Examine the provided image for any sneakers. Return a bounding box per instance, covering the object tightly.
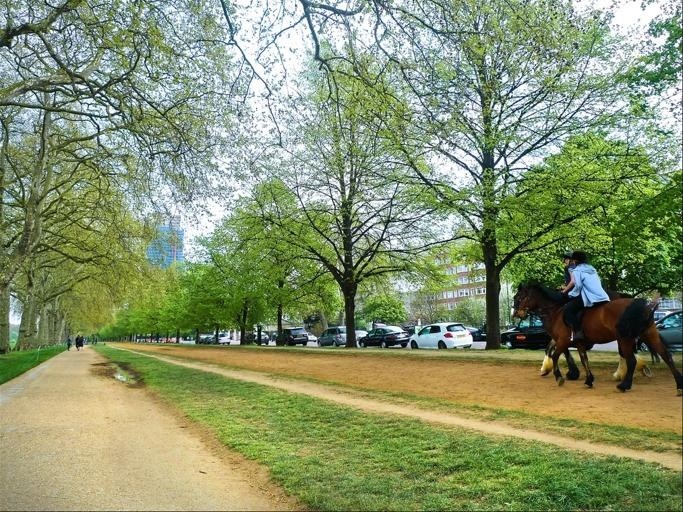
[573,330,585,339]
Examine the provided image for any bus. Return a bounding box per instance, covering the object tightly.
[136,334,151,344]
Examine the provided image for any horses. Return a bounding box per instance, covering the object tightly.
[512,276,683,396]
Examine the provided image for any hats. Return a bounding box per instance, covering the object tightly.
[563,251,586,260]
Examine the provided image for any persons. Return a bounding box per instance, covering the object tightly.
[561,251,576,286]
[63,334,71,351]
[74,334,80,351]
[560,250,611,342]
[80,335,83,347]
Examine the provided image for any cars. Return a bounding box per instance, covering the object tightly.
[637,308,683,352]
[193,332,231,345]
